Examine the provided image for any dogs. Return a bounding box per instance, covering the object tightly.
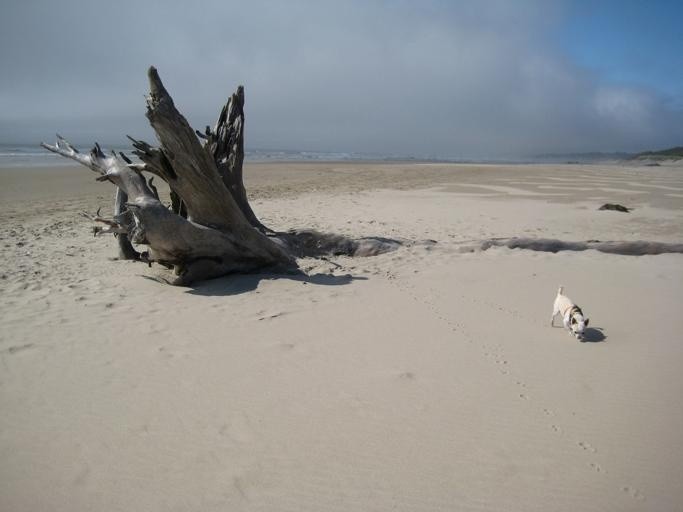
[550,285,589,340]
